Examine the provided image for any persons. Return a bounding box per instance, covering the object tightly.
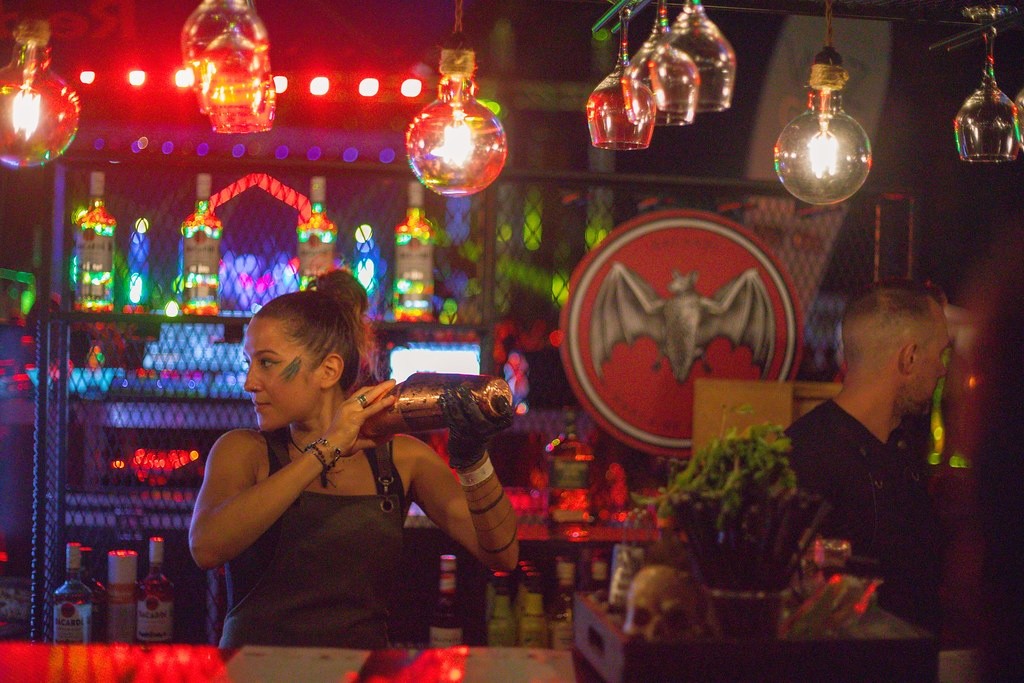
[774,279,948,602]
[189,268,519,647]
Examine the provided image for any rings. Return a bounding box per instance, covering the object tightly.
[355,394,367,404]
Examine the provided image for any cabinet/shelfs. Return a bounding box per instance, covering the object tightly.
[3,142,790,640]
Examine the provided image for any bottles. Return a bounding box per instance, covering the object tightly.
[180,173,222,315]
[73,171,115,315]
[483,553,610,651]
[52,537,176,645]
[392,181,434,322]
[428,554,465,649]
[549,408,591,525]
[296,176,339,294]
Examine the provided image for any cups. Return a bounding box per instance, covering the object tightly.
[1013,88,1024,152]
[359,372,513,439]
[701,583,792,639]
[180,0,276,133]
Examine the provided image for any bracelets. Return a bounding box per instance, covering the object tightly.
[305,438,344,488]
[459,457,494,487]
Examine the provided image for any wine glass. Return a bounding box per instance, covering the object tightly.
[586,0,737,152]
[953,4,1018,163]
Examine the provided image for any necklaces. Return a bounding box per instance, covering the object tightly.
[290,439,345,473]
